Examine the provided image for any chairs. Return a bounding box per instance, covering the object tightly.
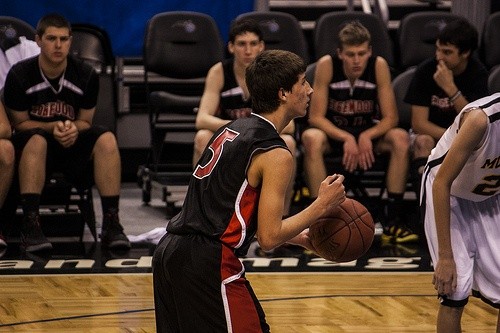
[0,16,118,242]
[139,12,500,220]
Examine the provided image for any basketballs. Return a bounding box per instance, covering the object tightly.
[309,198,375,262]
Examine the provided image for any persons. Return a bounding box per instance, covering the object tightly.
[152,50,345,333]
[0,100,15,259]
[301,21,418,242]
[420,92,500,333]
[2,15,131,252]
[403,15,489,178]
[194,21,296,246]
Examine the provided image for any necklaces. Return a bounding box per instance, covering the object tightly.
[40,67,66,94]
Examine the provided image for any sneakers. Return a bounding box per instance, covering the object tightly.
[102,214,132,250]
[303,248,319,256]
[21,212,52,253]
[381,223,418,242]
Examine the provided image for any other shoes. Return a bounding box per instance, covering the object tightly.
[0,227,8,247]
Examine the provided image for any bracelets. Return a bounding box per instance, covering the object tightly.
[448,90,461,101]
